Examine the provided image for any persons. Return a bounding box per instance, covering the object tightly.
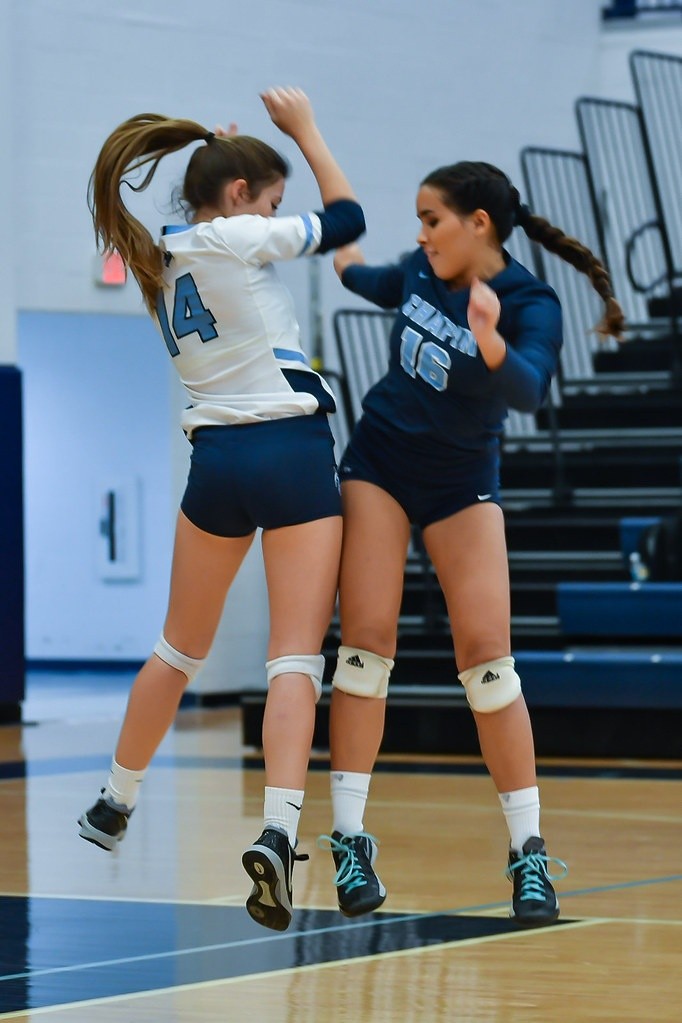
[75,85,366,933]
[317,160,627,926]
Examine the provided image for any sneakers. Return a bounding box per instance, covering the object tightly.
[505,836,567,928]
[77,788,135,851]
[316,831,386,918]
[242,825,309,932]
